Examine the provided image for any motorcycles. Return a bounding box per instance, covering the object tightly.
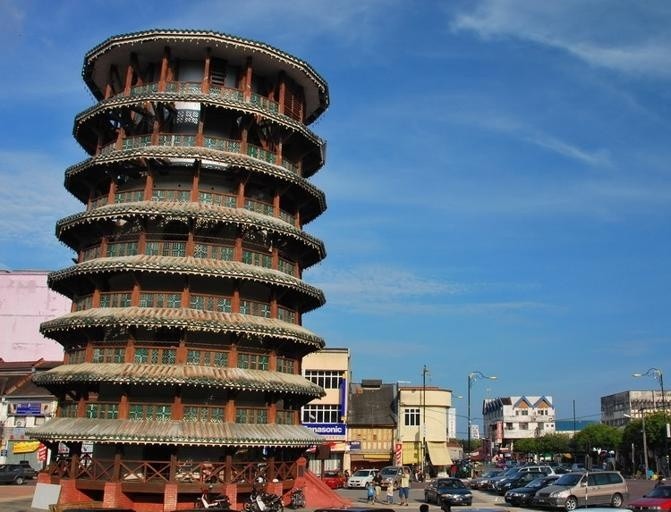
[243,481,307,512]
[194,485,232,509]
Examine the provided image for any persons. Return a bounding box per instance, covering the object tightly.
[345,466,423,506]
[419,504,429,512]
[441,500,452,512]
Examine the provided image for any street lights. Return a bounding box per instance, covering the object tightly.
[623,413,650,480]
[468,371,497,456]
[631,367,671,476]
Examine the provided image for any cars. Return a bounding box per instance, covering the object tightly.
[321,466,412,491]
[0,463,35,485]
[471,461,671,512]
[424,478,472,506]
